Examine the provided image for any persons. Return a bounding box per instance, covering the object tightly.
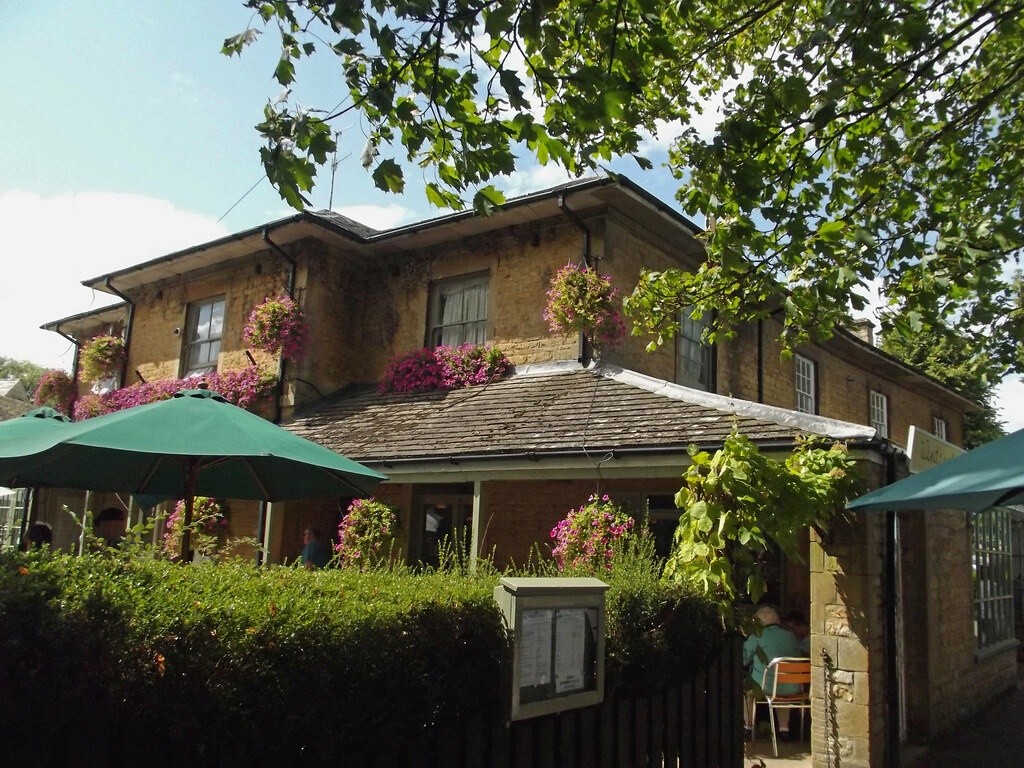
[302,526,327,569]
[93,508,126,550]
[785,611,810,657]
[742,606,802,738]
[23,522,53,550]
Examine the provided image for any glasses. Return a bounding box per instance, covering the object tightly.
[302,533,308,536]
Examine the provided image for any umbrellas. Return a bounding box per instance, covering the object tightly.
[0,407,72,444]
[0,377,391,559]
[846,428,1024,514]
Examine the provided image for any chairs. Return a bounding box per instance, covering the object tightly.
[751,657,812,757]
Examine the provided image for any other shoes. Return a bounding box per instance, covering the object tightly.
[743,728,751,741]
[778,730,791,741]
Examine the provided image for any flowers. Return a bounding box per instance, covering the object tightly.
[332,497,400,559]
[163,496,226,560]
[242,294,314,365]
[542,257,626,355]
[80,334,127,385]
[73,365,279,412]
[551,492,637,574]
[377,342,513,394]
[32,368,77,417]
